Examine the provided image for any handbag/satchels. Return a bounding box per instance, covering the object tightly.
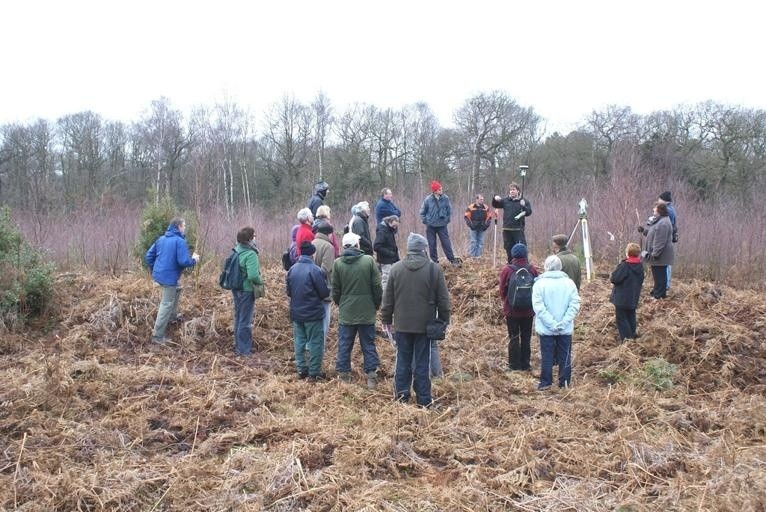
[640,250,650,261]
[426,318,446,340]
[253,285,265,298]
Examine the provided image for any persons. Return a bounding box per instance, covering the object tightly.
[463,193,492,257]
[637,202,675,300]
[492,182,533,263]
[498,242,540,372]
[531,254,582,391]
[229,226,266,357]
[419,180,457,265]
[284,182,403,391]
[380,232,450,409]
[551,233,582,293]
[658,191,679,291]
[608,242,645,344]
[143,218,202,343]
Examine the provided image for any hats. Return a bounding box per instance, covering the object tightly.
[319,223,333,234]
[660,191,674,202]
[552,234,569,245]
[431,180,441,193]
[407,233,427,251]
[299,241,316,255]
[543,254,563,270]
[511,244,528,257]
[342,232,361,248]
[316,181,328,192]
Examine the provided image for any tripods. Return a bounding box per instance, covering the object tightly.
[568,220,595,280]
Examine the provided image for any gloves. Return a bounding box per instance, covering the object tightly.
[638,226,644,232]
[552,322,565,331]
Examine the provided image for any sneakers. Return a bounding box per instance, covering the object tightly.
[298,371,326,383]
[368,369,393,380]
[151,337,170,344]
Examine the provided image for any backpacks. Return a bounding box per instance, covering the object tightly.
[282,245,295,270]
[507,263,534,307]
[219,248,249,290]
